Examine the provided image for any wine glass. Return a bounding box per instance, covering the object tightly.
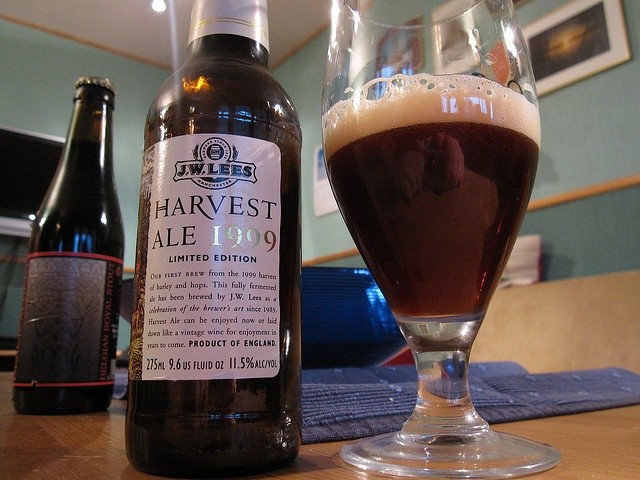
[320,0,561,479]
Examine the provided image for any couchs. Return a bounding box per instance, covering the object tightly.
[468,273,639,374]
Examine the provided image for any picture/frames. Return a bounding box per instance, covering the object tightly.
[521,1,630,98]
[374,18,423,90]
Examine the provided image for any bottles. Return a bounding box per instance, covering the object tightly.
[124,0,302,475]
[12,77,123,411]
